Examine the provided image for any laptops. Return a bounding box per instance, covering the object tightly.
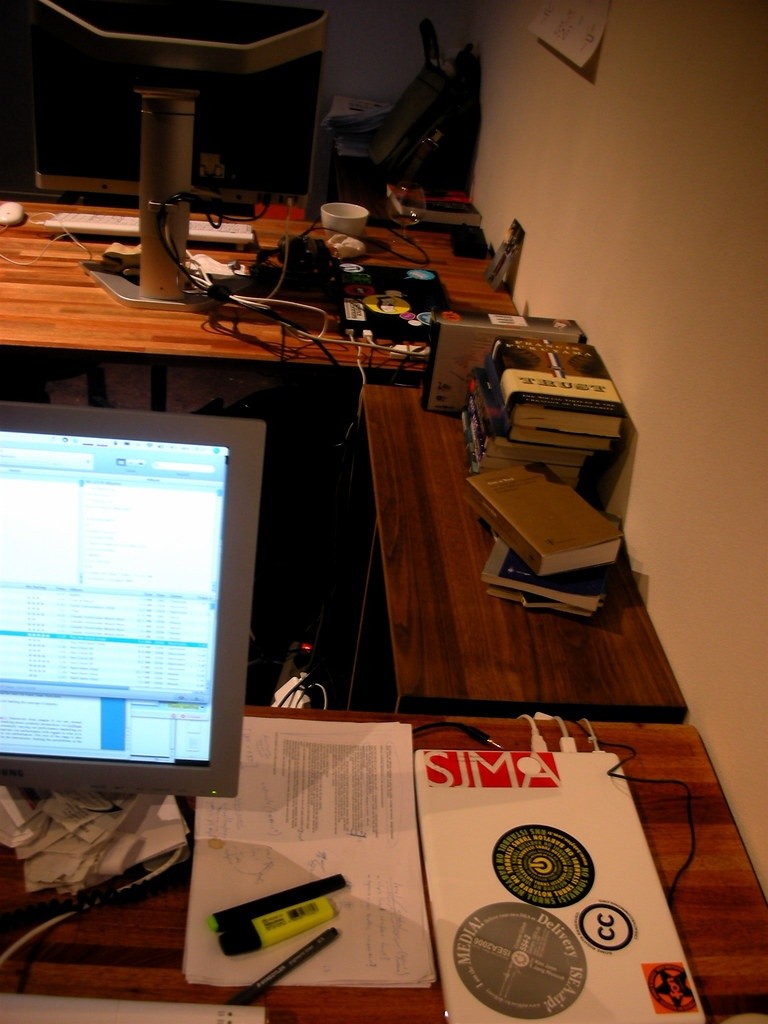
[336,264,453,340]
[410,749,706,1024]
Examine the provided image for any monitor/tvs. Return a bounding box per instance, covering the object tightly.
[27,0,329,311]
[0,402,266,799]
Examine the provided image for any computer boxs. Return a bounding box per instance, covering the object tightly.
[422,306,586,416]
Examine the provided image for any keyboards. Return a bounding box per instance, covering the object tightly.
[44,212,254,252]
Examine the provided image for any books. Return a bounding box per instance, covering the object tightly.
[387,181,482,227]
[460,333,630,618]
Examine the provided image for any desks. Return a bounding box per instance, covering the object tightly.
[345,383,687,719]
[0,706,768,1024]
[327,142,480,224]
[0,196,521,414]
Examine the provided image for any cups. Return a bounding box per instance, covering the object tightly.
[321,203,370,238]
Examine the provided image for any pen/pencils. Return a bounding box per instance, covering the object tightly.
[225,925,339,1005]
[207,874,346,932]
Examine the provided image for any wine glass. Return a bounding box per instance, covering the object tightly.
[386,182,426,248]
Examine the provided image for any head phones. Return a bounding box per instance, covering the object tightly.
[253,238,339,292]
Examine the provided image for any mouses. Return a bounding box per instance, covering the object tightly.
[0,202,24,226]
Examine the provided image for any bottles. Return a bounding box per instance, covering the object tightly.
[402,129,443,180]
